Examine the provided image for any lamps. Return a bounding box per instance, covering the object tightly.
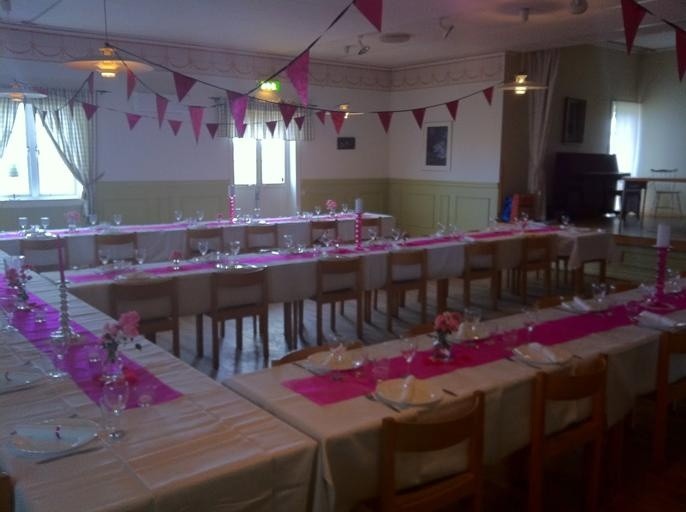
[494,6,549,96]
[356,34,371,56]
[1,80,47,104]
[568,1,589,14]
[438,16,456,39]
[62,0,155,79]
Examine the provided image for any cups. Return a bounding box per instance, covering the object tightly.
[135,382,156,408]
[33,306,47,323]
[86,342,102,361]
[11,255,24,273]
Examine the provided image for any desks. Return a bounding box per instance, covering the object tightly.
[620,175,685,230]
[2,210,685,511]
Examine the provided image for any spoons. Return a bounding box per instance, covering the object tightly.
[364,390,400,413]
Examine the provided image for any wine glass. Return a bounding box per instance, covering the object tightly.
[101,376,130,439]
[0,296,19,332]
[13,201,351,245]
[97,213,569,281]
[48,331,69,378]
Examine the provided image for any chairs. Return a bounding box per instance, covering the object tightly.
[648,167,682,218]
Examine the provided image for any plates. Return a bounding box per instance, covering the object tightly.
[6,418,101,454]
[305,269,686,407]
[0,366,46,391]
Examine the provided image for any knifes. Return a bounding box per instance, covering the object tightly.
[292,360,323,376]
[36,447,102,463]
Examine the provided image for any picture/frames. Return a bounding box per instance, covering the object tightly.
[420,120,452,172]
[560,95,586,145]
